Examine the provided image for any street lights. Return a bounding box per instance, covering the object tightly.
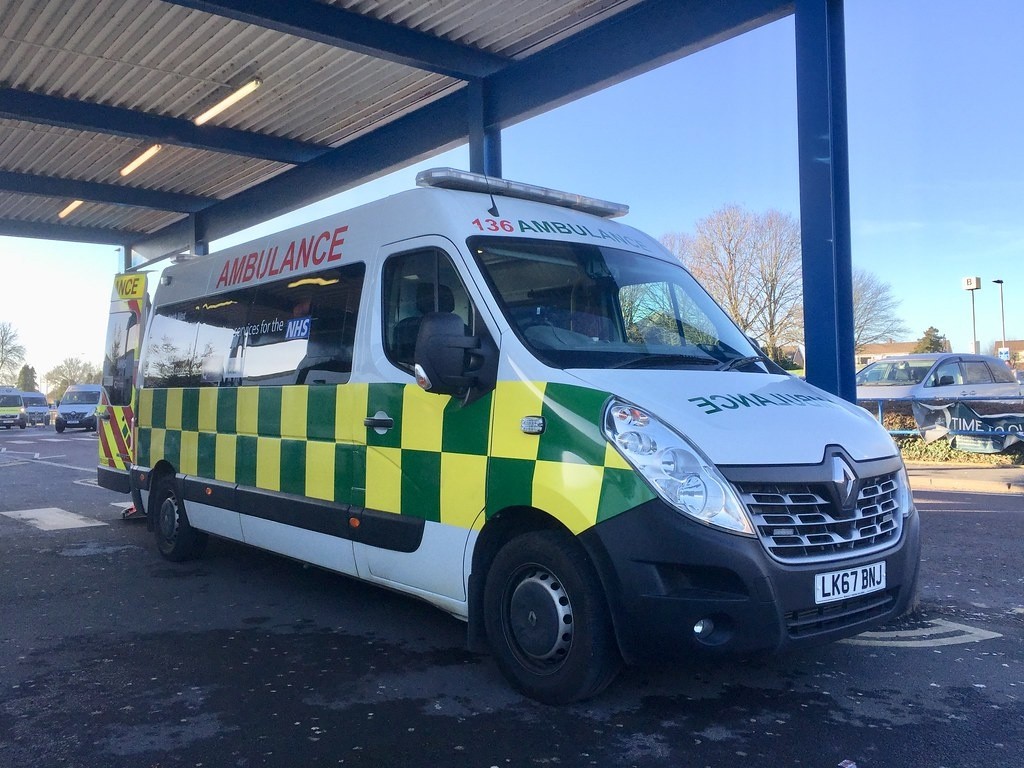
[993,279,1006,360]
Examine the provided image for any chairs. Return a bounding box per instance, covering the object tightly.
[894,370,911,380]
[936,366,954,378]
[513,300,563,327]
[73,396,79,401]
[393,282,474,378]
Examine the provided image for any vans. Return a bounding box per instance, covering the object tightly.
[20,391,51,426]
[54,384,102,433]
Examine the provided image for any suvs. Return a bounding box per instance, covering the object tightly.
[854,355,1024,439]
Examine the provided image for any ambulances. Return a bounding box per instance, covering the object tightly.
[93,164,923,707]
[1,385,27,429]
